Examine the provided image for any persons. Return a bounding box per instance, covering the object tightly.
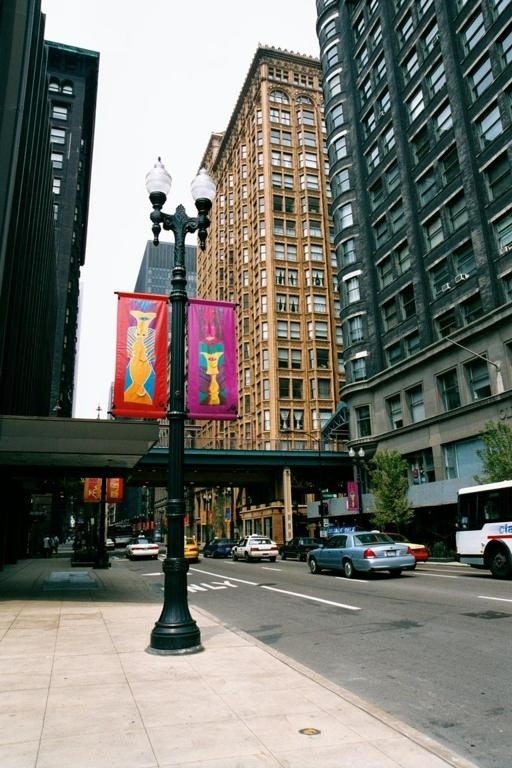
[43,536,60,557]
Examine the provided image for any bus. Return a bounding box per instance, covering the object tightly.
[454,479,512,579]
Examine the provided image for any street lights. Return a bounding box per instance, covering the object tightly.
[145,155,219,656]
[277,426,326,532]
[94,404,103,421]
[349,446,366,529]
[202,491,212,545]
[158,506,165,542]
